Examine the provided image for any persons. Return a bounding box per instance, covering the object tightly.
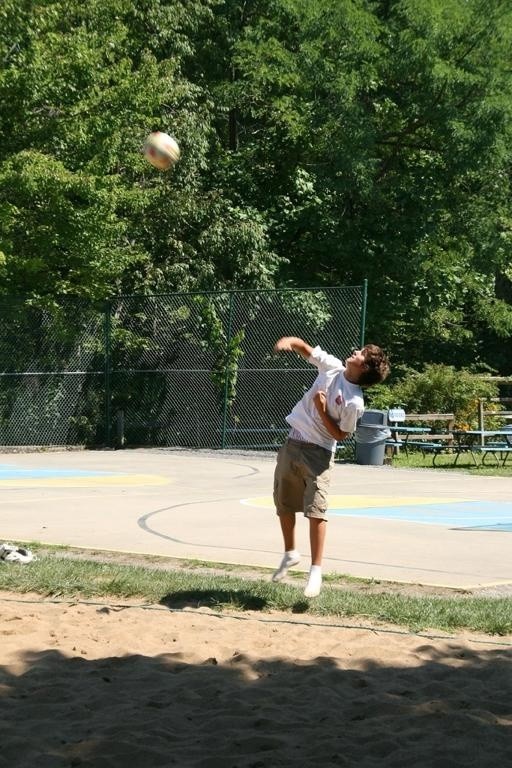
[271,336,390,598]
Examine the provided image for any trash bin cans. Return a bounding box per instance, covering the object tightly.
[354,410,391,465]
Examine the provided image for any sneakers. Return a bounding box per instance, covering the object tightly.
[0,542,33,564]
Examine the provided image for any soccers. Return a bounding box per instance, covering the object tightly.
[143,132,180,170]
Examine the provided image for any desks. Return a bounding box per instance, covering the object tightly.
[385,426,512,470]
